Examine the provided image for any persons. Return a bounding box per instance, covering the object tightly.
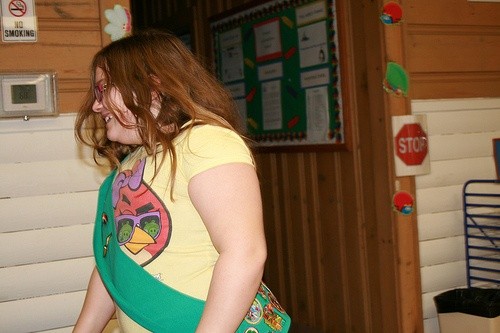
[72,28,292,333]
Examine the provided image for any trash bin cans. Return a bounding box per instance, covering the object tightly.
[433,287,500,333]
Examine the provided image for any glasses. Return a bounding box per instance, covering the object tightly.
[94,82,115,102]
